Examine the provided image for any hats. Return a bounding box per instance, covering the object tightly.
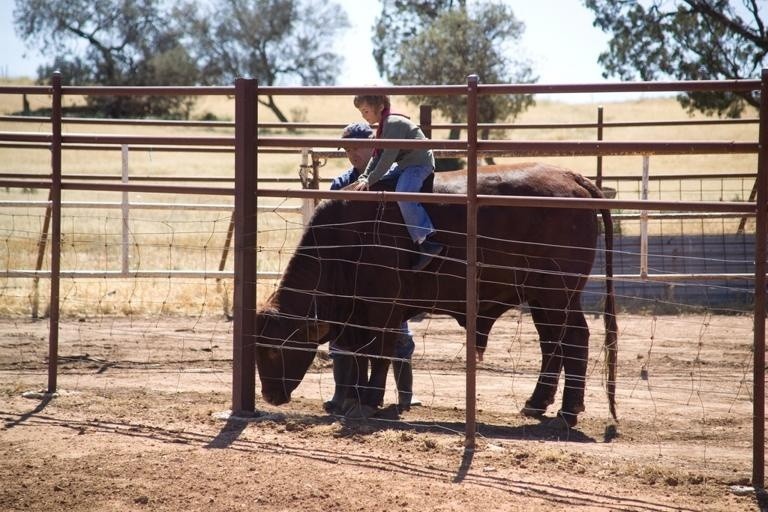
[338,122,376,150]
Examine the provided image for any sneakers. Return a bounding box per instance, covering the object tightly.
[412,239,443,272]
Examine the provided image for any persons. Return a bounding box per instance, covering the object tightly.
[324,122,416,412]
[353,94,446,272]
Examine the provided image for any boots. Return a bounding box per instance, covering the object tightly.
[323,357,354,413]
[387,359,413,413]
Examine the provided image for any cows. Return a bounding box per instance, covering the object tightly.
[256,161,619,430]
